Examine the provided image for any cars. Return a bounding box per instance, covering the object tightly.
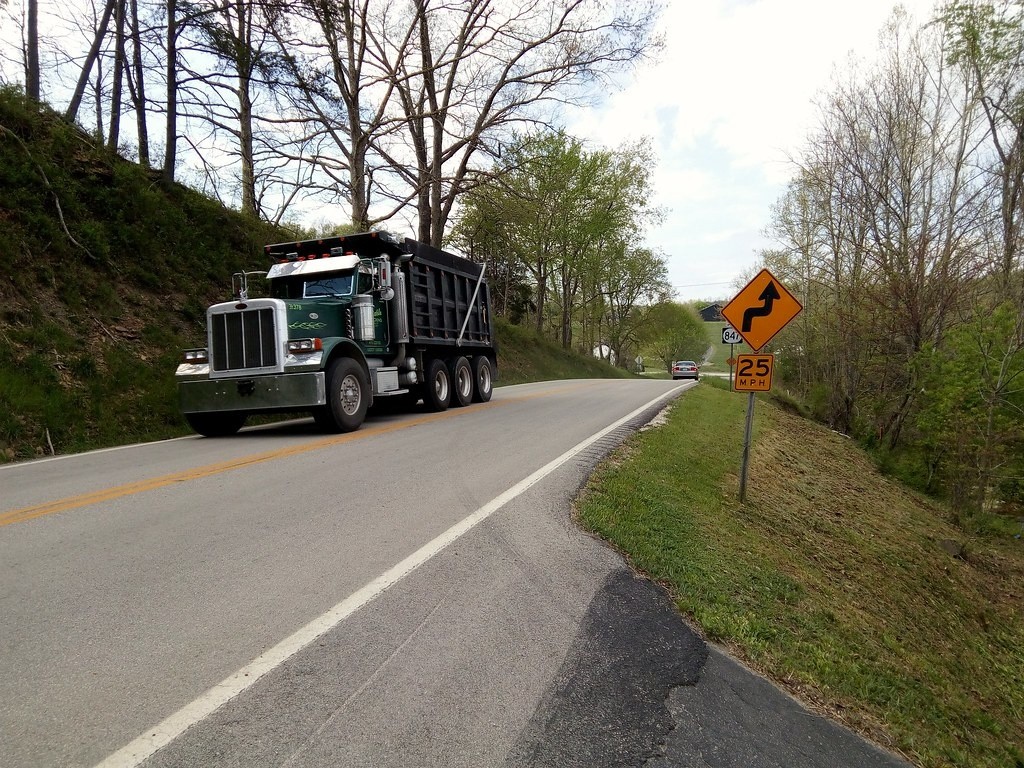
[672,360,700,381]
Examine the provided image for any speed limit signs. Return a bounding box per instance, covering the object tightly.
[734,354,774,393]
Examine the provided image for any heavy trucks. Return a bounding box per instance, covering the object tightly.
[170,230,500,439]
